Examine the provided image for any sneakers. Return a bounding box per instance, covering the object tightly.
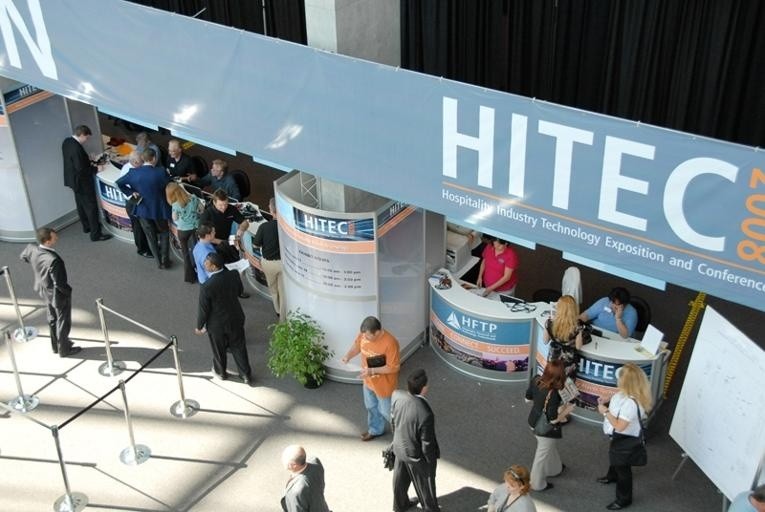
[361,433,384,441]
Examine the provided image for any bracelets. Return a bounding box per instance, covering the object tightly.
[602,411,610,416]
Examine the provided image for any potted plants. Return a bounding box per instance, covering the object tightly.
[260,305,337,389]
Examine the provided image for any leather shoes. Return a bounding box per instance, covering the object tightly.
[598,478,609,483]
[91,234,111,241]
[607,501,622,509]
[212,368,226,379]
[240,293,248,298]
[137,248,171,268]
[544,482,553,491]
[60,347,81,356]
[409,497,418,506]
[239,375,249,383]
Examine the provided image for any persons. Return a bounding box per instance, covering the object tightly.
[580,287,639,338]
[115,148,175,269]
[281,442,329,512]
[542,296,581,425]
[18,227,81,358]
[203,188,249,297]
[61,124,112,240]
[187,158,242,198]
[476,238,517,297]
[340,316,400,442]
[254,197,283,317]
[525,358,577,492]
[390,367,442,512]
[165,181,205,281]
[192,222,219,284]
[726,481,765,512]
[194,251,254,385]
[135,131,159,160]
[120,151,152,256]
[597,362,650,510]
[485,462,537,512]
[163,137,195,184]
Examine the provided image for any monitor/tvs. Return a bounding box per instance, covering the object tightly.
[499,292,525,305]
[181,182,203,198]
[110,159,123,170]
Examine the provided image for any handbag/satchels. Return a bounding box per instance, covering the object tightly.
[382,443,395,471]
[609,437,646,466]
[535,411,553,436]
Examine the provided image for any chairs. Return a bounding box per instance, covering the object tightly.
[531,289,561,304]
[629,296,653,331]
[156,144,251,203]
